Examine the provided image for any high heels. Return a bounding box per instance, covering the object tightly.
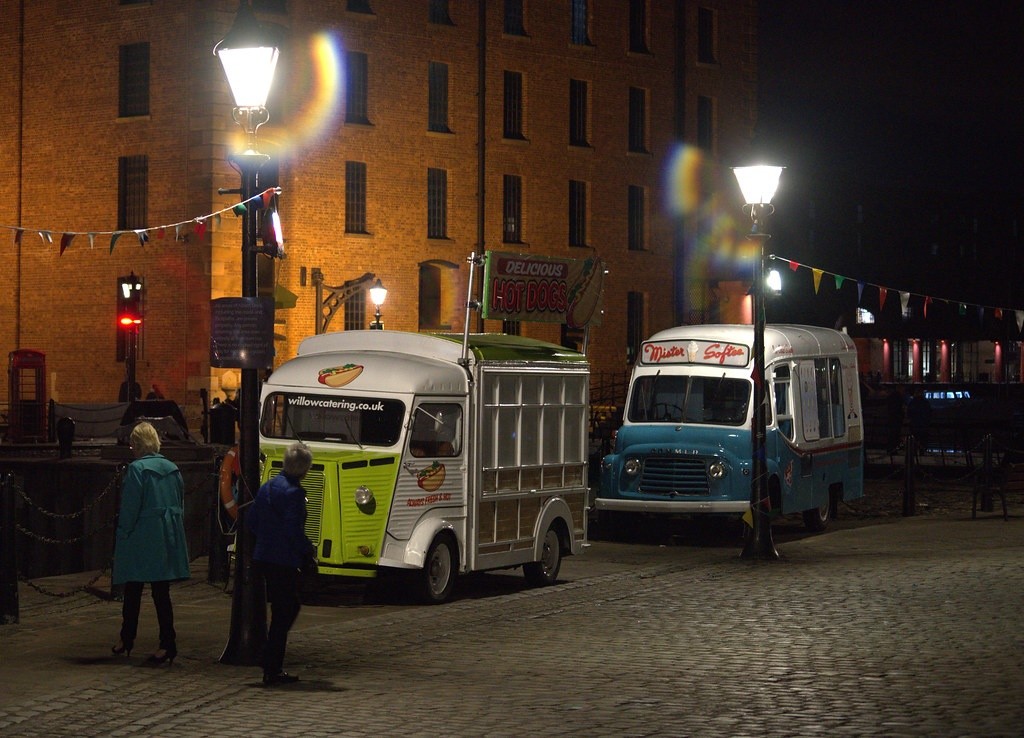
[111,641,134,656]
[147,648,177,664]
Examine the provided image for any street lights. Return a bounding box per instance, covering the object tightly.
[215,11,293,669]
[726,138,792,565]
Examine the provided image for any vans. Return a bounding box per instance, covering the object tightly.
[594,323,865,544]
[227,328,592,606]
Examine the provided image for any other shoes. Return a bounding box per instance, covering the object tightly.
[263,673,275,686]
[273,671,299,687]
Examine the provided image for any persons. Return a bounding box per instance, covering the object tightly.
[886,384,906,449]
[859,370,883,385]
[245,443,316,685]
[110,423,191,668]
[146,384,166,401]
[907,387,932,456]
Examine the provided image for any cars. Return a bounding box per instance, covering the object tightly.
[857,379,888,401]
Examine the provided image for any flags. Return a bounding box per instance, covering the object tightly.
[788,260,1024,334]
[13,187,276,255]
